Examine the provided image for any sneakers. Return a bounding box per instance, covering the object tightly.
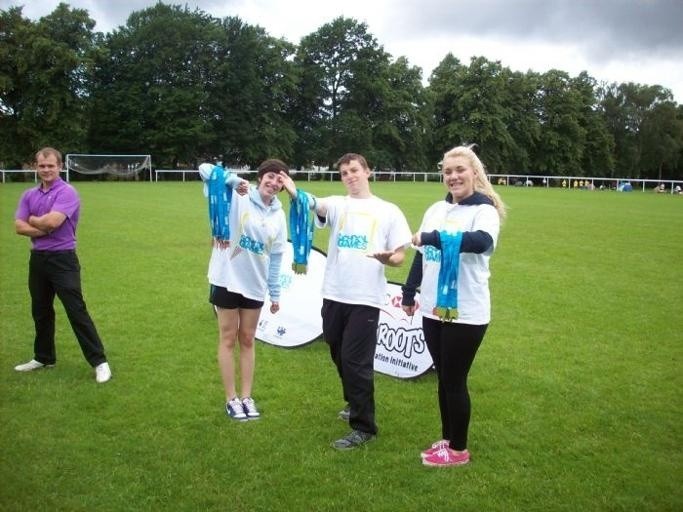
[95,362,111,383]
[240,397,260,419]
[14,359,54,371]
[336,403,351,423]
[421,447,469,467]
[420,439,450,457]
[329,430,376,450]
[224,397,248,421]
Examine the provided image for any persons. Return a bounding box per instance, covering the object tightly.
[277,151,414,451]
[197,158,290,422]
[653,184,667,193]
[674,185,682,193]
[495,178,590,188]
[400,144,511,467]
[12,147,112,384]
